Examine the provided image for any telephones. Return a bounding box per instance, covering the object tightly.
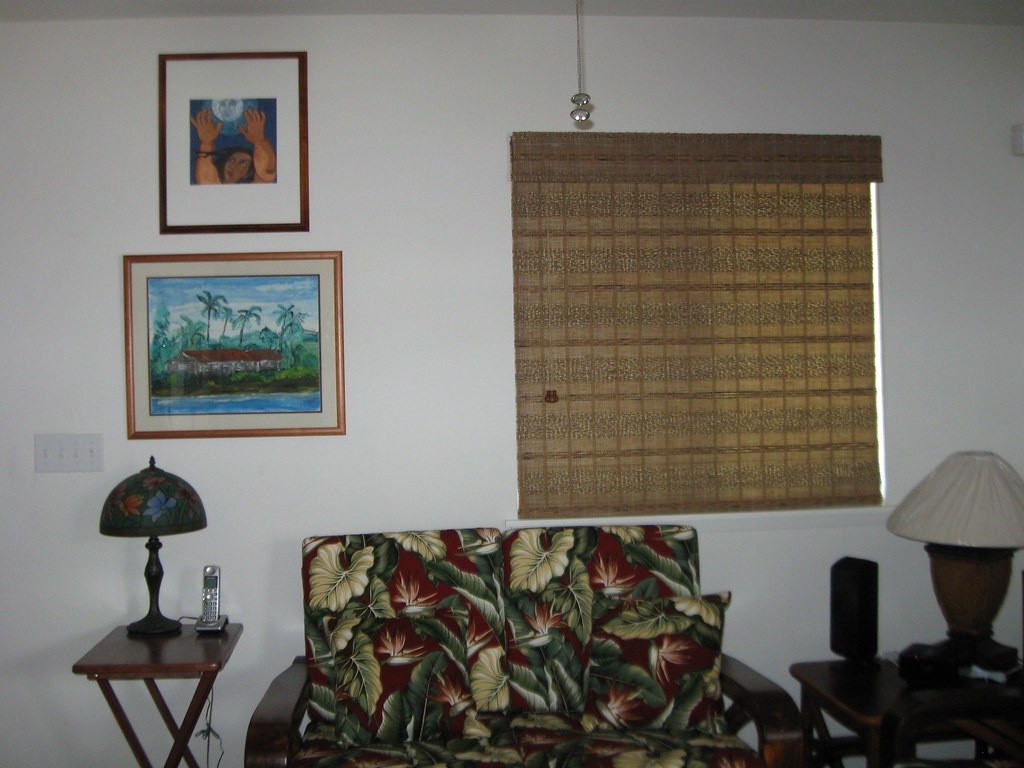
[194,564,229,634]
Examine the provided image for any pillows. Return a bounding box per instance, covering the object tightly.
[582,590,731,739]
[322,604,492,751]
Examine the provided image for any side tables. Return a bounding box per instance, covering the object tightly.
[789,650,1024,768]
[72,623,243,768]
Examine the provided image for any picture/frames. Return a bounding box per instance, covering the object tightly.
[159,50,309,235]
[123,250,346,440]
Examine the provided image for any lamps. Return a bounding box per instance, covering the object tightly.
[99,455,207,636]
[886,451,1024,671]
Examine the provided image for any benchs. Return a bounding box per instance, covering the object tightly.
[244,524,804,768]
